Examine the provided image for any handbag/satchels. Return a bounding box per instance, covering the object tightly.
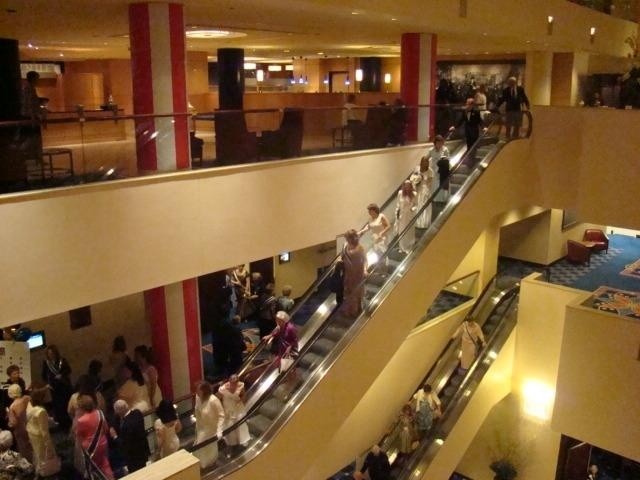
[328,271,344,293]
[477,337,483,347]
[265,334,295,373]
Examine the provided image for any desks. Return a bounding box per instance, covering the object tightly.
[26,147,74,182]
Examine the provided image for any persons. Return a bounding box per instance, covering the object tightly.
[336,229,370,319]
[352,442,392,479]
[25,70,49,149]
[215,373,252,448]
[448,97,489,169]
[341,94,361,145]
[0,326,163,480]
[356,202,390,274]
[394,179,419,256]
[436,78,498,136]
[425,133,451,206]
[209,264,304,387]
[364,100,387,124]
[618,67,639,109]
[450,316,487,370]
[191,379,225,470]
[385,402,421,455]
[409,155,434,229]
[153,399,182,461]
[495,76,530,138]
[405,384,444,433]
[587,464,603,480]
[386,98,406,115]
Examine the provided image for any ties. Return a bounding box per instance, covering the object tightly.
[512,89,515,97]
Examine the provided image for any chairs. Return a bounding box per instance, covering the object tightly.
[214,107,303,167]
[567,229,609,267]
